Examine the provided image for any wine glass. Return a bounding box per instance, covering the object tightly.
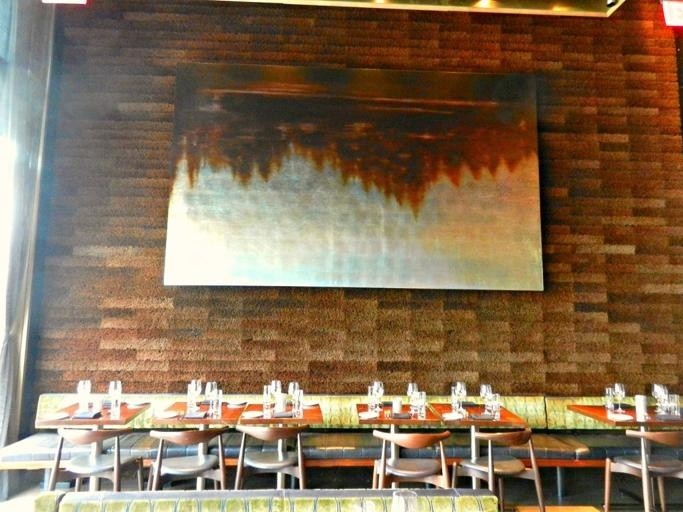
[606,384,683,416]
[74,379,305,420]
[369,379,501,422]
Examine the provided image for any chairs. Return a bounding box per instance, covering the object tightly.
[372,428,547,511]
[603,428,682,512]
[46,427,303,491]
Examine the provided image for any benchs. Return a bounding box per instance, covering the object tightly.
[34,489,499,511]
[546,395,683,458]
[1,396,575,469]
